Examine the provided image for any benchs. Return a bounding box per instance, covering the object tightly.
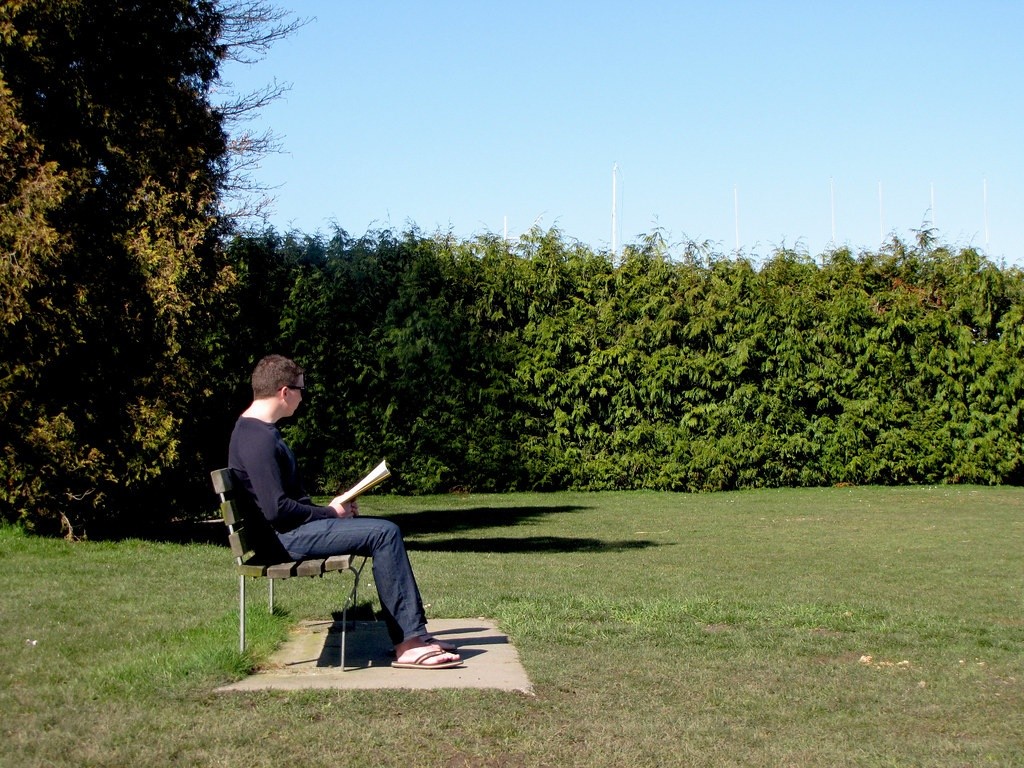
[211,468,367,672]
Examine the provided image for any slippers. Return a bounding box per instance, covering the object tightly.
[390,648,465,669]
[425,638,458,650]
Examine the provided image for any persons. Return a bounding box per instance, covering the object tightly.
[228,354,464,670]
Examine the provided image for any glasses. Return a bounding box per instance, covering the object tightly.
[278,385,306,399]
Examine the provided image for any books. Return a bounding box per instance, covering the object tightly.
[328,459,390,505]
